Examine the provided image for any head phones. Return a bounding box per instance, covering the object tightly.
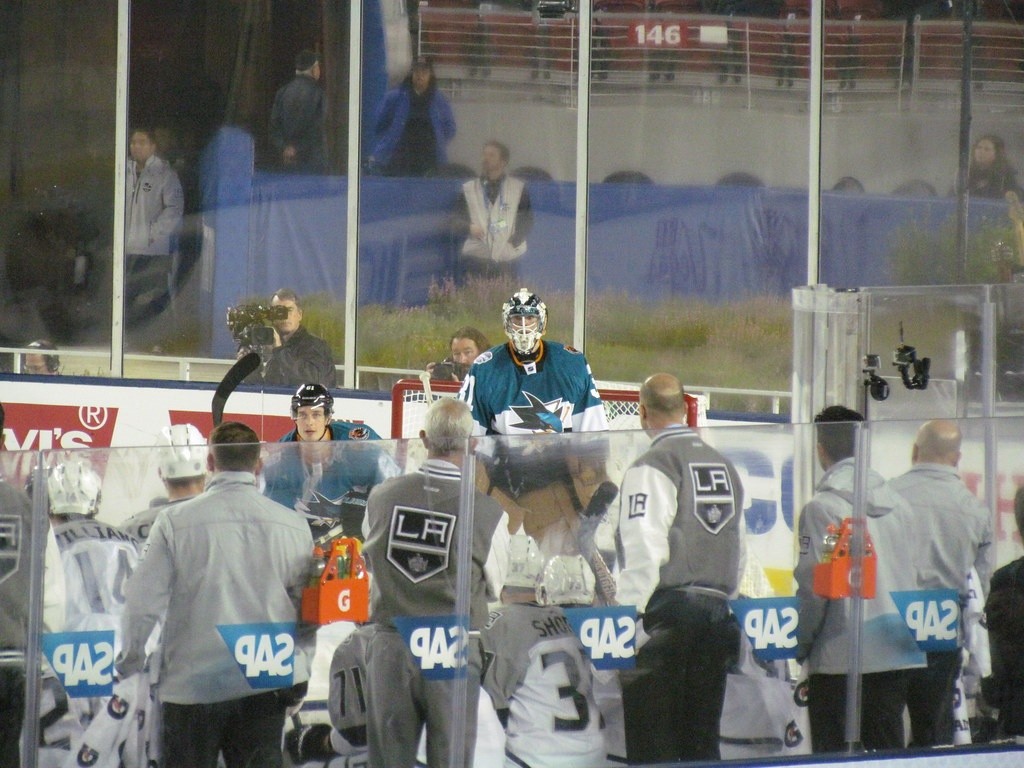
[35,338,60,373]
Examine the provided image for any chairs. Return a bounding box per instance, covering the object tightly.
[468,0,954,90]
[429,164,938,200]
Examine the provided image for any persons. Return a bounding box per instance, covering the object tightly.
[984,488,1024,746]
[267,50,329,176]
[456,289,611,559]
[427,325,491,382]
[887,420,994,746]
[362,396,508,768]
[125,127,184,354]
[0,403,370,768]
[447,141,531,283]
[264,383,400,547]
[616,374,751,765]
[482,535,607,768]
[238,288,336,388]
[363,57,456,176]
[793,406,929,754]
[24,340,60,375]
[948,133,1024,202]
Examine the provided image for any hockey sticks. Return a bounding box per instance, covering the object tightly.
[574,479,618,609]
[211,351,262,427]
[419,371,433,407]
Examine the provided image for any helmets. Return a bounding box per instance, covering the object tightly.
[157,423,209,478]
[292,384,334,414]
[501,288,548,356]
[47,463,101,515]
[536,555,596,605]
[503,534,542,588]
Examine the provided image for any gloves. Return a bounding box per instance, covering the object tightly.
[340,491,369,542]
[285,723,337,765]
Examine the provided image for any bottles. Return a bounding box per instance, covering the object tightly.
[308,536,366,589]
[822,524,872,561]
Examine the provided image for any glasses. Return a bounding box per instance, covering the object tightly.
[23,360,51,374]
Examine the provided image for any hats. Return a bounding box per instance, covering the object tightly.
[295,50,320,71]
[411,55,432,69]
